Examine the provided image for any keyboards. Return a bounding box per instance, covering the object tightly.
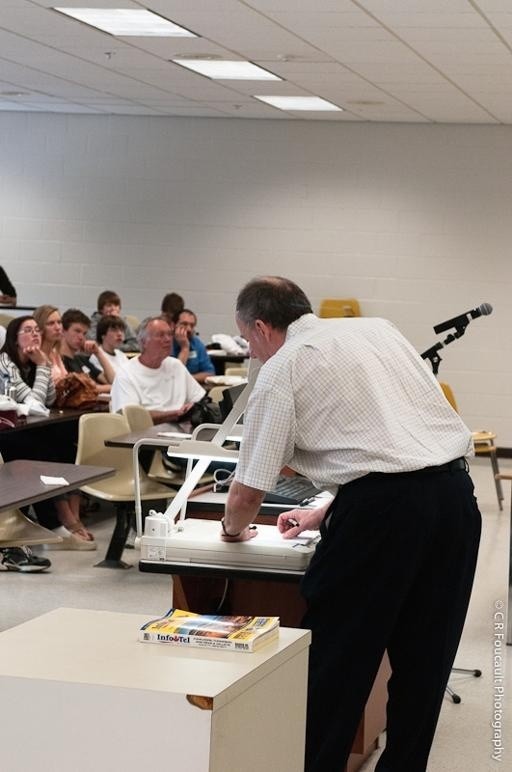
[263,474,320,505]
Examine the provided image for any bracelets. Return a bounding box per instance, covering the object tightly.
[219,517,242,537]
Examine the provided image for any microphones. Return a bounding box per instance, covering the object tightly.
[433,302,493,334]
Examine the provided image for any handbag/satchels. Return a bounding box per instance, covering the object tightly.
[190,402,222,428]
[55,372,98,409]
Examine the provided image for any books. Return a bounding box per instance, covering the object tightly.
[137,606,281,652]
[206,375,242,387]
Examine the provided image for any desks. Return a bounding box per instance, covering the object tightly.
[1,606,311,772]
[0,406,110,534]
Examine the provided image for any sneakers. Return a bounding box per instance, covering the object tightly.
[0,547,51,572]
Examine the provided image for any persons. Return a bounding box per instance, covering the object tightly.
[212,273,483,771]
[0,288,217,575]
[2,266,21,308]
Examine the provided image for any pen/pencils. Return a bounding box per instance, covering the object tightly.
[287,518,300,527]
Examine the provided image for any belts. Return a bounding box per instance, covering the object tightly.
[429,459,466,470]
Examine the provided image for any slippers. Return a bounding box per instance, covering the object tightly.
[67,523,94,541]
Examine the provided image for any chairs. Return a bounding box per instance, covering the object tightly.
[438,381,506,512]
[74,407,214,570]
